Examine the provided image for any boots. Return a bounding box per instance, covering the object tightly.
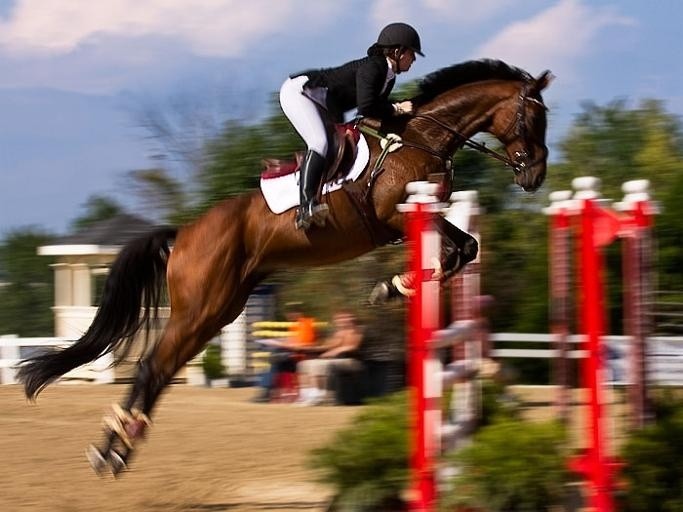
[295,150,329,233]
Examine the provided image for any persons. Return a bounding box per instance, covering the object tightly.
[279,23,424,230]
[248,302,364,403]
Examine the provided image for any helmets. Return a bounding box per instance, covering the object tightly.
[376,23,425,56]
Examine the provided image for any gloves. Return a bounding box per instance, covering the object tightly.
[391,101,415,115]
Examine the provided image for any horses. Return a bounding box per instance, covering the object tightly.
[11,56,556,482]
